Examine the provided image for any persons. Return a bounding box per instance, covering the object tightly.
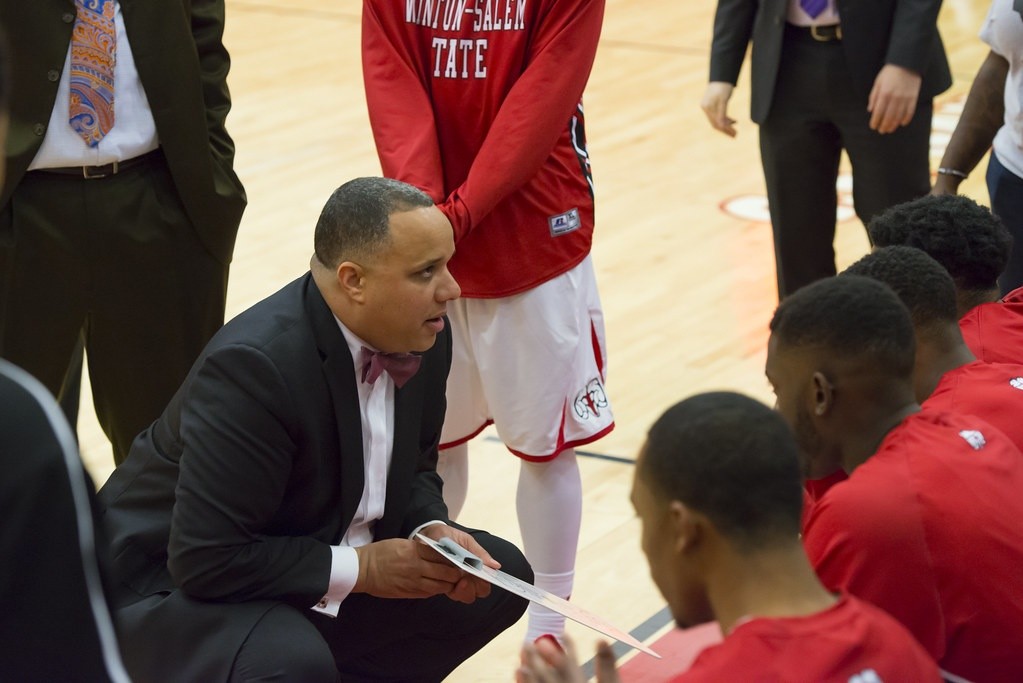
[507,392,945,683]
[0,0,249,490]
[767,276,1023,682]
[701,0,955,299]
[867,194,1023,367]
[1,359,135,683]
[930,0,1023,258]
[361,0,605,645]
[837,246,1023,453]
[94,178,533,683]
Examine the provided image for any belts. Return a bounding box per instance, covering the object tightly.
[41,147,164,179]
[780,19,844,43]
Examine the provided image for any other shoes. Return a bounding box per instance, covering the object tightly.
[533,633,565,655]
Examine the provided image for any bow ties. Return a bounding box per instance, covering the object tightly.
[360,346,423,388]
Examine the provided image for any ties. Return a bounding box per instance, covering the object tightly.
[68,0,115,147]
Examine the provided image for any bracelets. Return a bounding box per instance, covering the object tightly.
[937,167,967,177]
